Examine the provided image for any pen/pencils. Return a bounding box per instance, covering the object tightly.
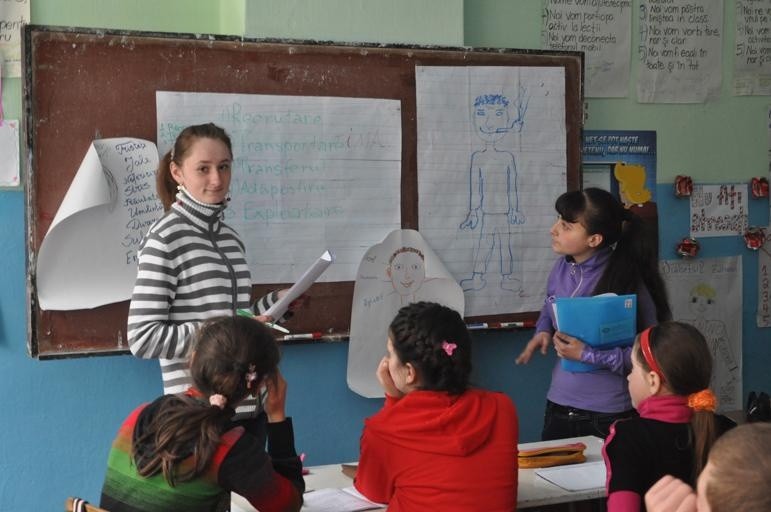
[235,308,290,335]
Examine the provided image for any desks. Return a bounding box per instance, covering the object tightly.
[229,434,606,512]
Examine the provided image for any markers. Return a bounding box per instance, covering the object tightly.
[496,322,533,328]
[465,323,488,330]
[284,332,321,340]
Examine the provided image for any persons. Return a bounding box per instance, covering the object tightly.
[647,423,767,511]
[126,123,311,396]
[100,317,305,512]
[352,302,517,512]
[515,187,660,442]
[602,322,738,511]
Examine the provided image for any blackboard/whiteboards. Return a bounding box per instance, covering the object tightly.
[20,24,584,360]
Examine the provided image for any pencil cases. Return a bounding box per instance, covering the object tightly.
[516,442,588,470]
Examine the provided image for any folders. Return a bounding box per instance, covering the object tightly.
[556,295,637,373]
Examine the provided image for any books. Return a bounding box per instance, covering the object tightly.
[536,460,607,492]
[302,487,388,512]
